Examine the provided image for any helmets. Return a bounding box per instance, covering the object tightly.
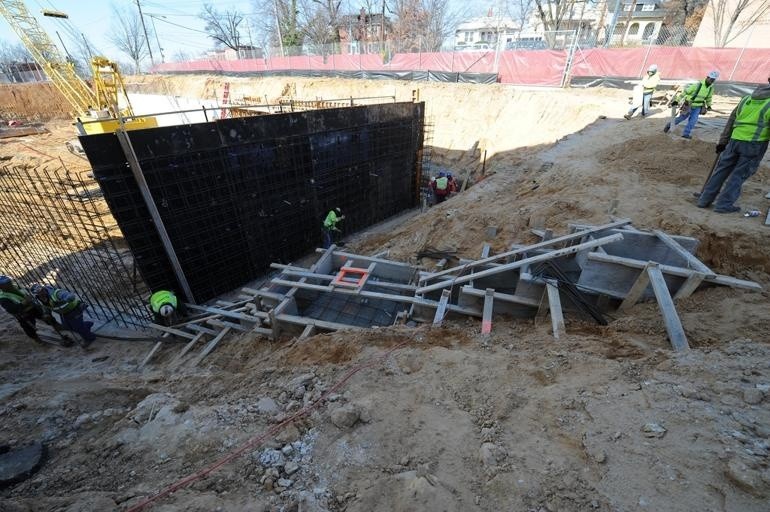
[647,65,657,75]
[708,71,718,79]
[31,285,45,297]
[0,276,10,284]
[440,172,451,176]
[160,305,173,317]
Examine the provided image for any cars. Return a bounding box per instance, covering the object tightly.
[454,38,591,53]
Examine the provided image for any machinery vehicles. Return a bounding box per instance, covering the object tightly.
[0,1,159,162]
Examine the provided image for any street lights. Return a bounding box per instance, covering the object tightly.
[151,14,168,62]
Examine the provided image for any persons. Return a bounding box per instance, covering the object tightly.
[149,289,180,326]
[431,171,452,202]
[30,282,95,349]
[663,71,718,138]
[0,276,59,347]
[446,173,458,192]
[323,205,346,245]
[698,78,769,214]
[625,64,660,119]
